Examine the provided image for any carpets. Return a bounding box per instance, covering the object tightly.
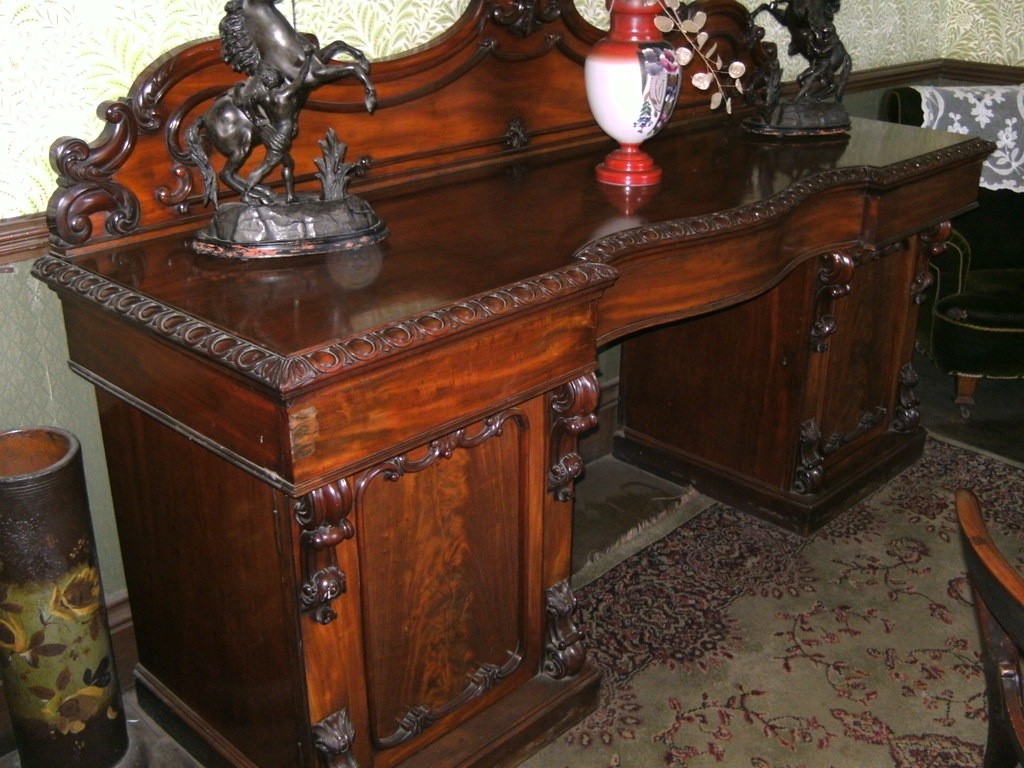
[517,430,1024,768]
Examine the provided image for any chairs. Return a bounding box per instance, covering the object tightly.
[956,488,1024,768]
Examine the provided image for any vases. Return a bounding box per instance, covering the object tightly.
[585,0,682,186]
[0,428,129,768]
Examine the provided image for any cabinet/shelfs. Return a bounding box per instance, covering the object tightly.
[28,0,997,768]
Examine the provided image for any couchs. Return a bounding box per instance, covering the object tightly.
[880,84,1024,418]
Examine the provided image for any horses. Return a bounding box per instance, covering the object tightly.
[745,0,853,105]
[182,0,380,212]
